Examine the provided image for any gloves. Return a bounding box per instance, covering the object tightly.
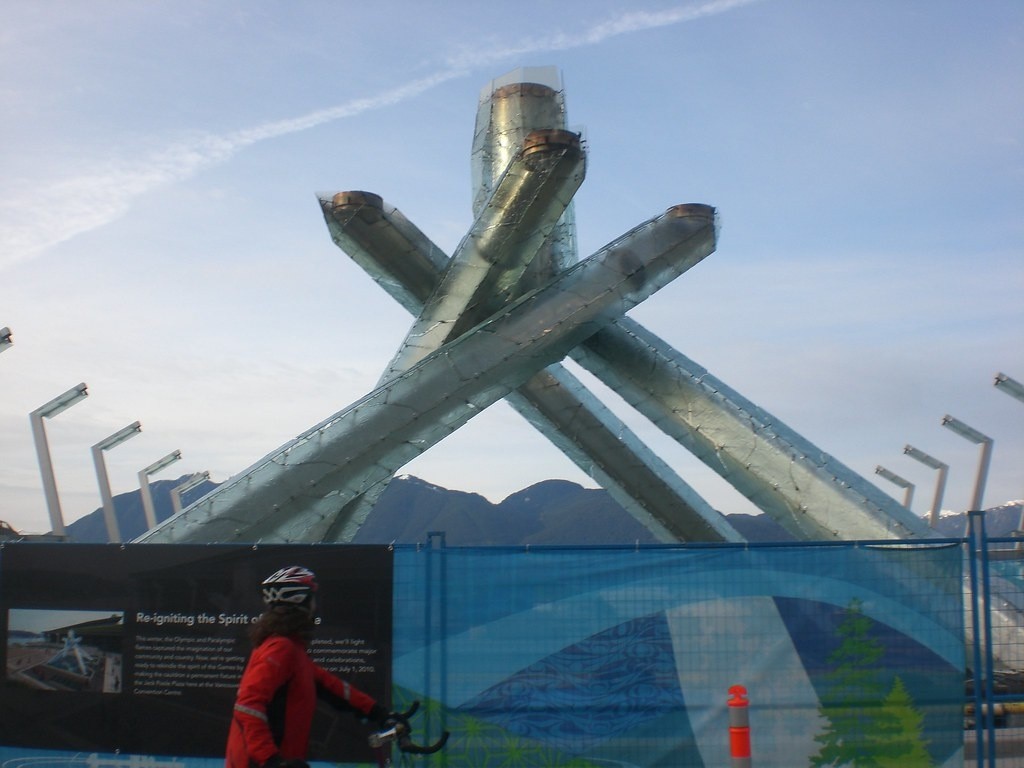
[263,753,288,768]
[367,703,391,724]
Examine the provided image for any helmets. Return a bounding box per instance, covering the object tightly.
[261,565,319,604]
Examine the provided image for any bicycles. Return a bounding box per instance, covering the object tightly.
[368,701,450,768]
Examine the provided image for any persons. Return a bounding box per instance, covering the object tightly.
[226,566,412,768]
